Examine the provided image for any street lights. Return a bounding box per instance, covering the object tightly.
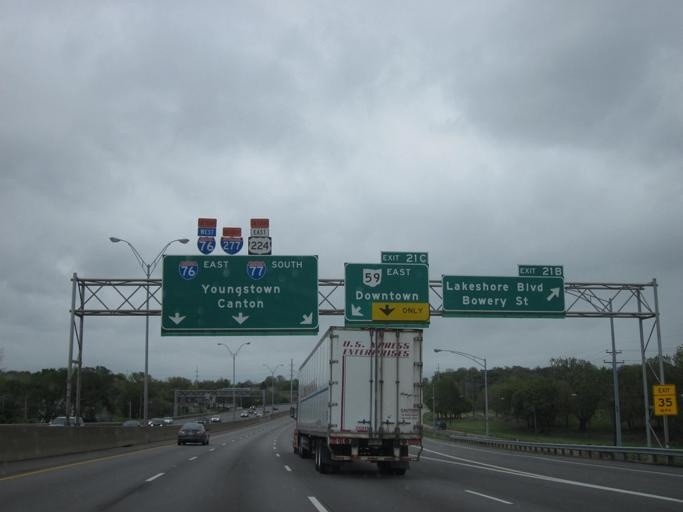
[107,231,191,427]
[433,346,491,438]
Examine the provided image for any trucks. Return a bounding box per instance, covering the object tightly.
[286,323,426,475]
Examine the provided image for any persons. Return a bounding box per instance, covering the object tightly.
[436,418,446,430]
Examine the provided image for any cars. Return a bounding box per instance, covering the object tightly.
[145,417,168,427]
[121,419,145,428]
[46,415,86,428]
[174,422,211,447]
[211,403,280,424]
[162,416,175,425]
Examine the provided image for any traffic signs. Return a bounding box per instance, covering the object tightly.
[260,362,284,414]
[343,250,430,326]
[441,265,565,319]
[197,216,218,255]
[161,254,319,335]
[246,216,274,255]
[220,225,246,255]
[215,340,251,422]
